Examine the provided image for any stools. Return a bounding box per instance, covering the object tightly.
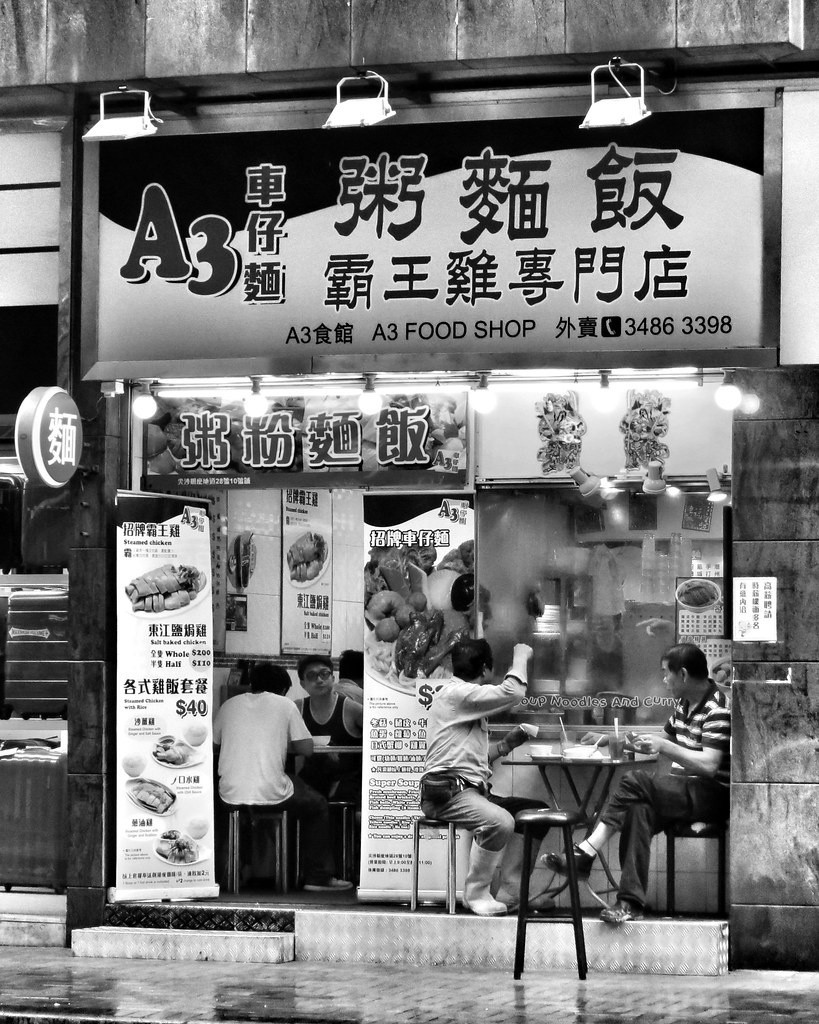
[514,807,587,982]
[220,800,358,897]
[409,817,474,914]
[663,822,727,917]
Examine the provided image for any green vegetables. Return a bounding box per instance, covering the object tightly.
[363,542,436,601]
[171,564,194,592]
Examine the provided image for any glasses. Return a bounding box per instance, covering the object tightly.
[304,669,332,680]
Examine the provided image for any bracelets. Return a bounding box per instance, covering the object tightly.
[497,740,508,757]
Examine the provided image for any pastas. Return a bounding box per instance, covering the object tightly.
[366,628,453,690]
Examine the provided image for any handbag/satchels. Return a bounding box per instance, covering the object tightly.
[422,773,467,802]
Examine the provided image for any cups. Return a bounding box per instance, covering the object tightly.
[639,533,692,601]
[609,733,625,760]
[560,731,576,754]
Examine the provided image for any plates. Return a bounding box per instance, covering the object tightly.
[524,753,562,759]
[365,655,417,697]
[153,830,210,865]
[533,605,561,639]
[285,533,330,587]
[121,573,208,618]
[126,778,182,817]
[532,678,588,693]
[151,735,205,768]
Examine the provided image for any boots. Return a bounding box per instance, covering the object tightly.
[494,833,557,911]
[463,837,507,916]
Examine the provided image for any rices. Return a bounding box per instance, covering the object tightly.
[185,721,206,738]
[123,750,146,768]
[186,817,207,839]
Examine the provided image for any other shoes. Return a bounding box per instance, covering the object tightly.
[600,900,644,924]
[303,877,353,891]
[541,844,596,881]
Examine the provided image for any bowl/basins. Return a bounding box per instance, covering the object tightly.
[674,579,721,613]
[123,763,146,777]
[529,744,553,756]
[312,736,332,745]
[186,824,208,840]
[182,728,207,747]
[710,656,732,693]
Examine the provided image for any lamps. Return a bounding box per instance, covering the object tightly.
[323,67,426,127]
[570,467,601,497]
[643,461,666,494]
[578,55,678,129]
[83,86,197,142]
[706,467,727,503]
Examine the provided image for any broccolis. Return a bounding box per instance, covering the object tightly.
[439,540,477,578]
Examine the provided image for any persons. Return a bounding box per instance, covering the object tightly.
[567,611,675,724]
[420,639,552,916]
[213,649,364,891]
[540,643,731,924]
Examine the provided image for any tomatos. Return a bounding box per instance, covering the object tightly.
[367,590,406,620]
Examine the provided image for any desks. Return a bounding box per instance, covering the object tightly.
[500,751,658,912]
[290,746,363,887]
